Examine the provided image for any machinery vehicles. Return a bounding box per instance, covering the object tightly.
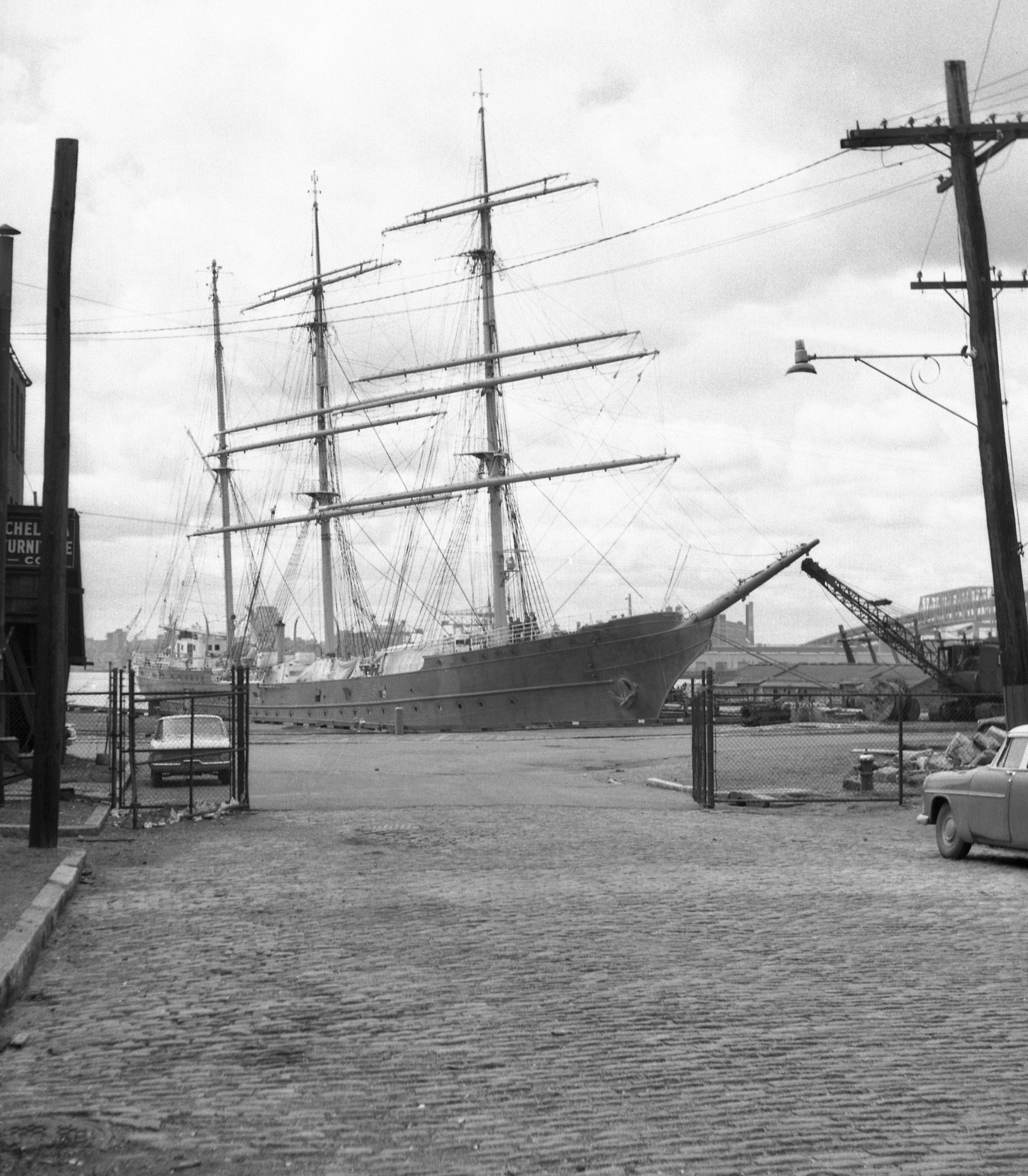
[802,557,1003,720]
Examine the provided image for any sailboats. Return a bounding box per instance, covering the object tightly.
[127,65,823,748]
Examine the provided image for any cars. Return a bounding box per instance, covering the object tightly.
[916,723,1028,862]
[144,713,232,787]
